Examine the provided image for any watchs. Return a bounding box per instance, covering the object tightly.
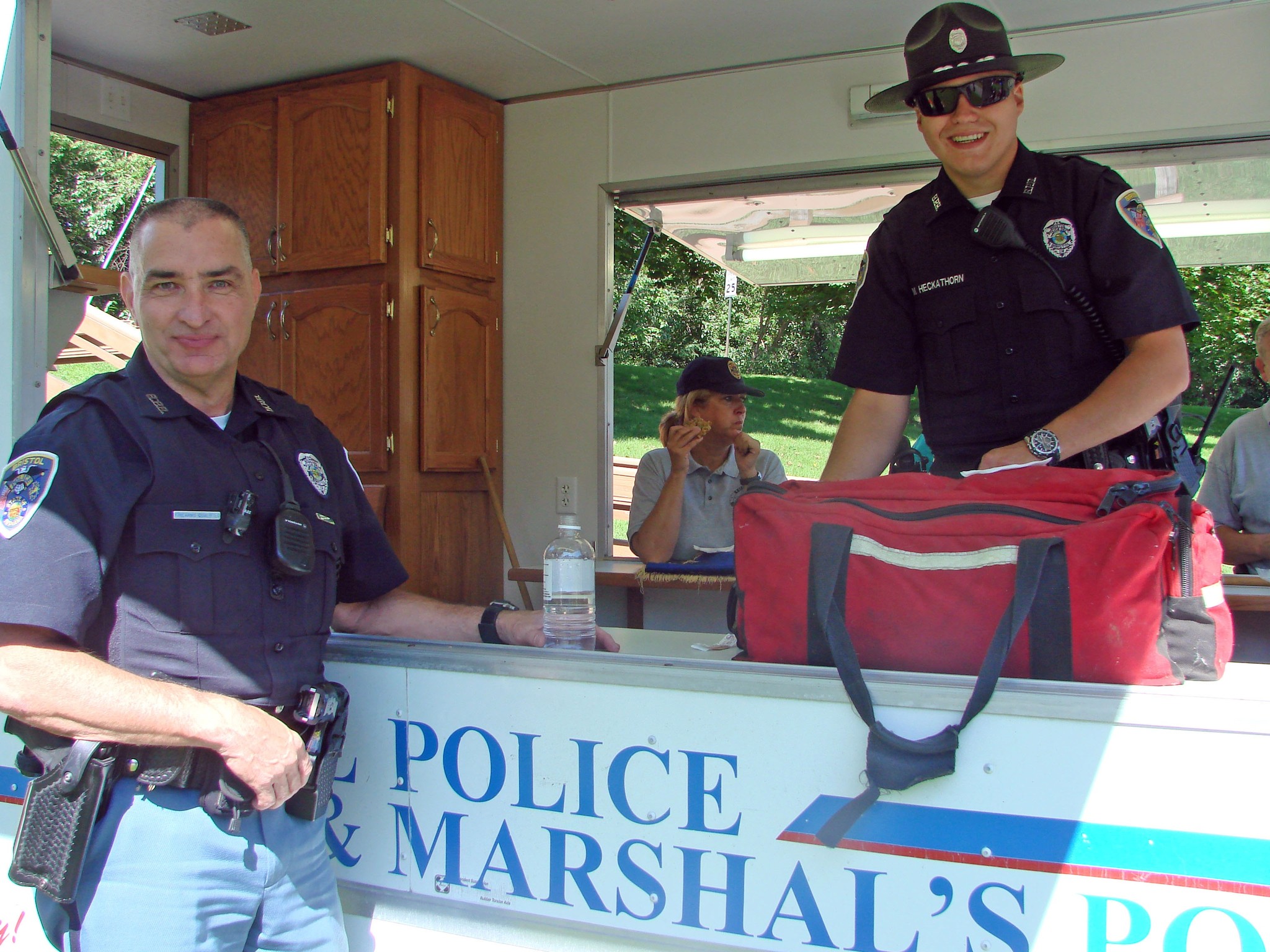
[739,471,763,486]
[1023,428,1062,465]
[478,600,520,643]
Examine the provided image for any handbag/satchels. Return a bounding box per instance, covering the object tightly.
[726,472,1235,791]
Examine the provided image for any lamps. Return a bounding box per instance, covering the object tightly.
[726,216,1270,262]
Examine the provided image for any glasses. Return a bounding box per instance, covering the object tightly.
[909,73,1024,118]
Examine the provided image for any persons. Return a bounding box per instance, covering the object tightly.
[627,354,788,565]
[815,3,1204,482]
[1195,315,1270,582]
[0,197,621,952]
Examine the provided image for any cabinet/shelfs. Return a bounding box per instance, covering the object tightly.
[184,60,505,608]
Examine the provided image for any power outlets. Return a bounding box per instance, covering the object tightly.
[556,475,578,515]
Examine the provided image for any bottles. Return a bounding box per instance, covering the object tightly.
[542,515,598,652]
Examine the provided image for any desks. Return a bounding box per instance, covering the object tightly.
[505,559,1270,629]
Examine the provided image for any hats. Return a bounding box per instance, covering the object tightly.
[866,2,1065,114]
[674,356,765,399]
